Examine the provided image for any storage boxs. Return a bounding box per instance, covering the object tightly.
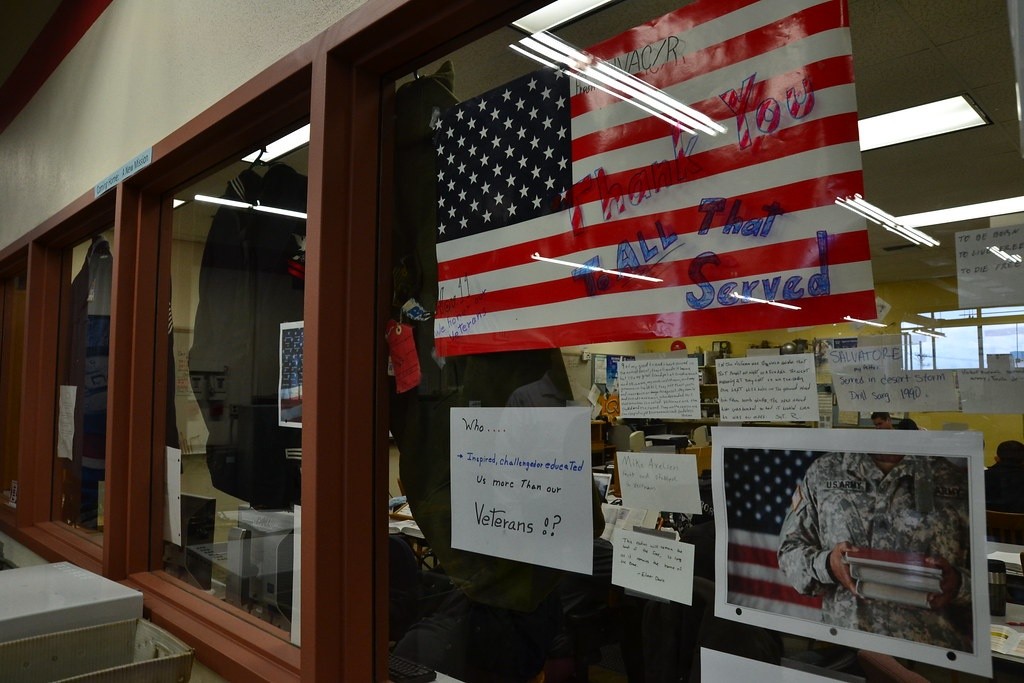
[0,561,195,683]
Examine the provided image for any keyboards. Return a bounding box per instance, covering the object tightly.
[388,653,437,683]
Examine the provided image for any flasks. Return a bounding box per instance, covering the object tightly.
[988,559,1006,616]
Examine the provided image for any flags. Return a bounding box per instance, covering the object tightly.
[435,0,878,359]
[725,447,975,652]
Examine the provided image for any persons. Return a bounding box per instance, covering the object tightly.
[493,329,616,512]
[868,410,1024,544]
[779,452,974,655]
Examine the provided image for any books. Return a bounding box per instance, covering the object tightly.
[845,550,944,609]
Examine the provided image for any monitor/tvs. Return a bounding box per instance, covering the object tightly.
[160,492,216,590]
[226,504,294,631]
